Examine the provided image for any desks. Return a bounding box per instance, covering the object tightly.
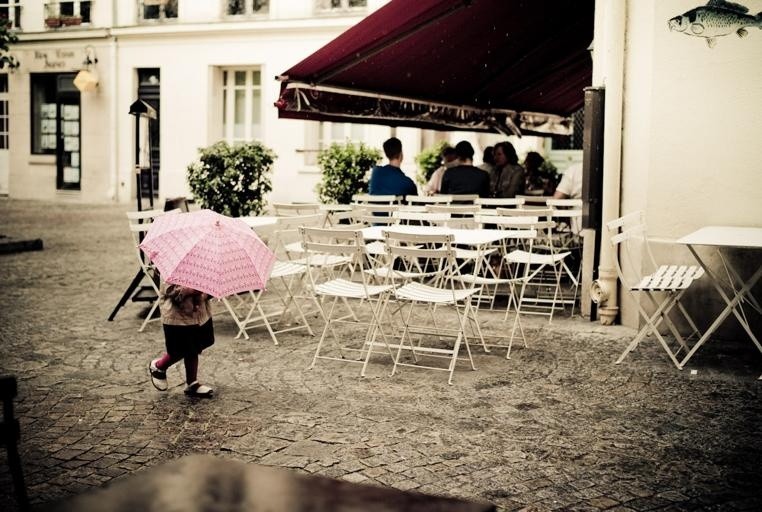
[676,226,761,368]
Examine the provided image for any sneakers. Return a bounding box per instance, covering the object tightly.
[148,359,170,391]
[185,381,213,395]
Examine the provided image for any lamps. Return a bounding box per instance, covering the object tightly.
[73,43,101,95]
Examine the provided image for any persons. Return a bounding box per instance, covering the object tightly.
[149,274,215,397]
[366,137,585,276]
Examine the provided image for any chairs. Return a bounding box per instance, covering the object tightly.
[107,190,585,386]
[607,210,702,370]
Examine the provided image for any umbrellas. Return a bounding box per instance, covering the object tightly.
[137,210,279,300]
